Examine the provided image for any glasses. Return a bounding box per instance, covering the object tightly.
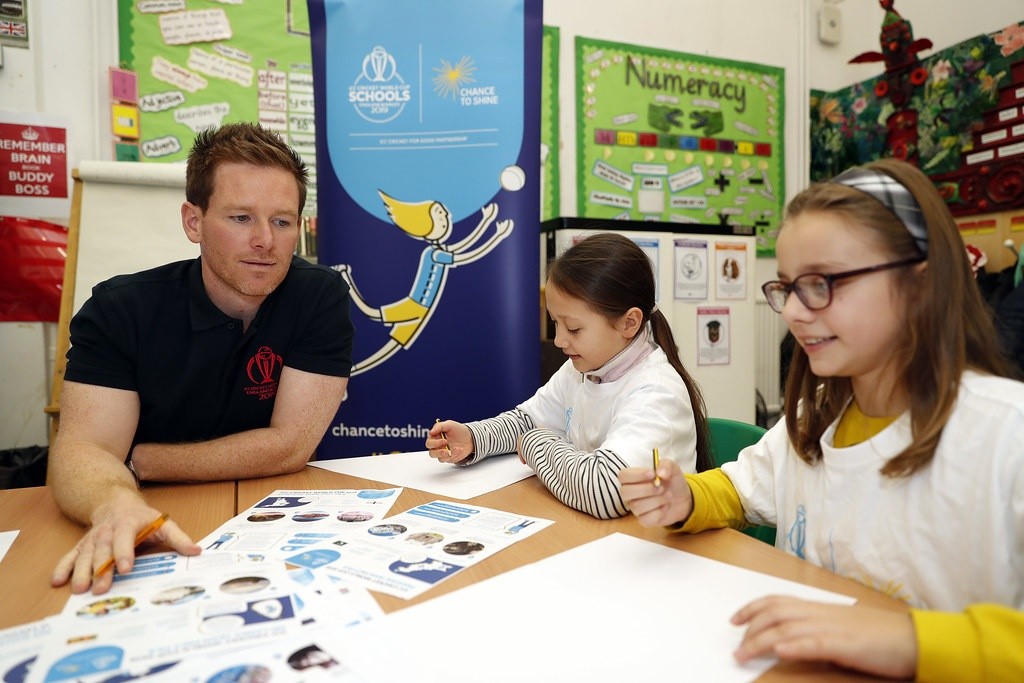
[761,255,930,314]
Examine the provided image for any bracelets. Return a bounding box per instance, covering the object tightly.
[125,443,138,488]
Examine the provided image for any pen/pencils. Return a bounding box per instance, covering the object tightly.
[652,448,661,488]
[436,418,452,458]
[90,511,170,582]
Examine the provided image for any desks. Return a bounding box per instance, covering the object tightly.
[0,465,920,683]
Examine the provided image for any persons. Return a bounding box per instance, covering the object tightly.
[618,159,1024,683]
[425,233,716,520]
[49,123,353,594]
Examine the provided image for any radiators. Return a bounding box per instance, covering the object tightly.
[755,299,788,415]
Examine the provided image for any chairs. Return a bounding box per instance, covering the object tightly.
[701,417,777,549]
